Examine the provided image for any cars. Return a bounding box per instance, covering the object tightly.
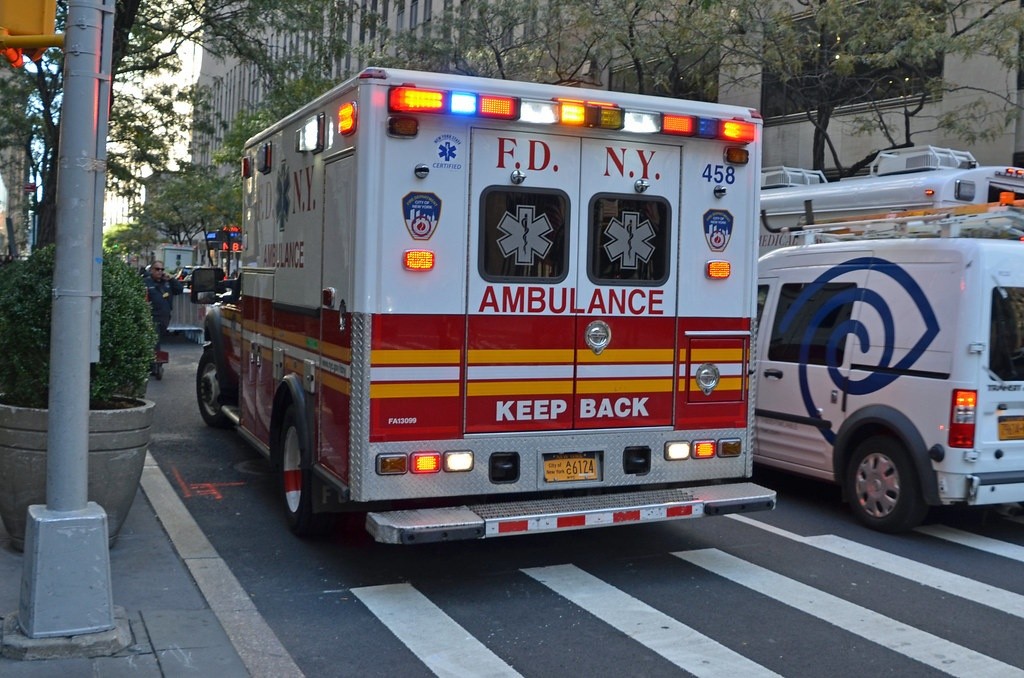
[171,263,226,289]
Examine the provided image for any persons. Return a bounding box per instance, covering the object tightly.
[144,261,184,380]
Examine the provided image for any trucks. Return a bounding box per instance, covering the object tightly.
[152,242,195,277]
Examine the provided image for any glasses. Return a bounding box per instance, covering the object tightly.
[151,267,165,271]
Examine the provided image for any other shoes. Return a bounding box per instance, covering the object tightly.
[151,363,158,374]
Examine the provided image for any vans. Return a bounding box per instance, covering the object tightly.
[754,143,1023,534]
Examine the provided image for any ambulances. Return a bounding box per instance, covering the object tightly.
[190,66,778,546]
